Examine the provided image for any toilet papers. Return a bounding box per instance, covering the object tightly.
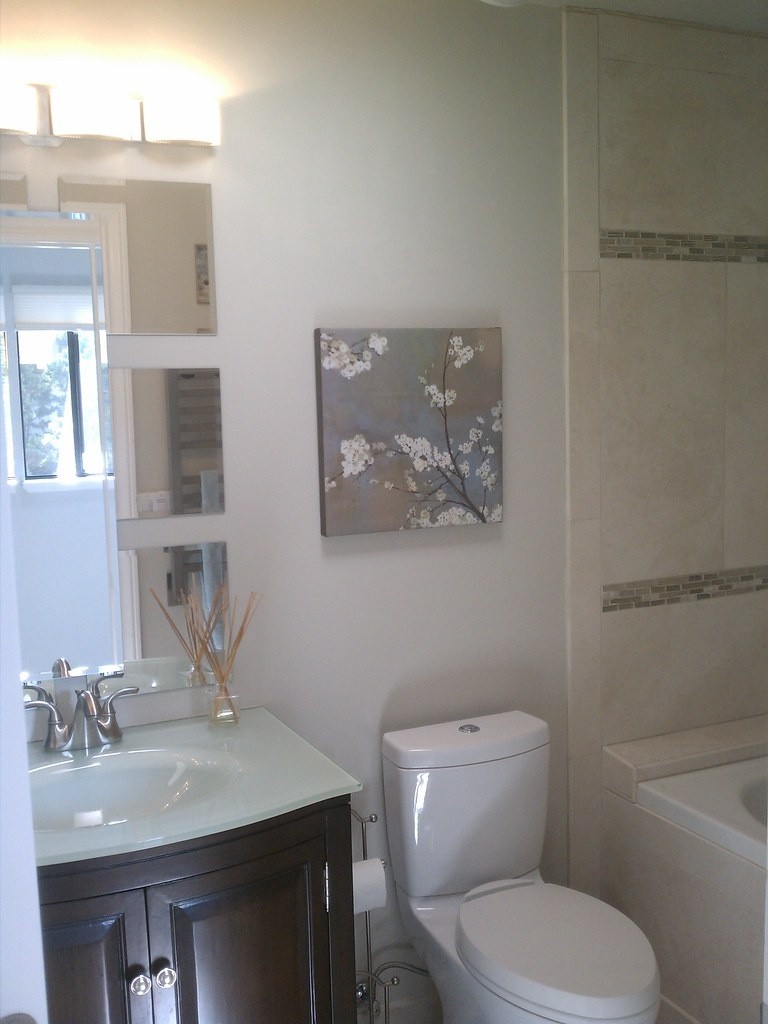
[352,857,387,916]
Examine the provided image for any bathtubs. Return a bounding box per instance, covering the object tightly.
[636,753,768,1024]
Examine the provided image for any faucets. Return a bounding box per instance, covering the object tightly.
[72,689,102,749]
[51,657,72,678]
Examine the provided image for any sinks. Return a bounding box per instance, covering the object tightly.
[28,749,242,832]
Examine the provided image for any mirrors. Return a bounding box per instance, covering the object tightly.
[0,178,221,709]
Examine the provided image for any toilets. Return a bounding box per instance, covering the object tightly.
[380,709,662,1024]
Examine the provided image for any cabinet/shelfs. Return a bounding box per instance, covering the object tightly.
[104,334,222,661]
[36,791,358,1024]
